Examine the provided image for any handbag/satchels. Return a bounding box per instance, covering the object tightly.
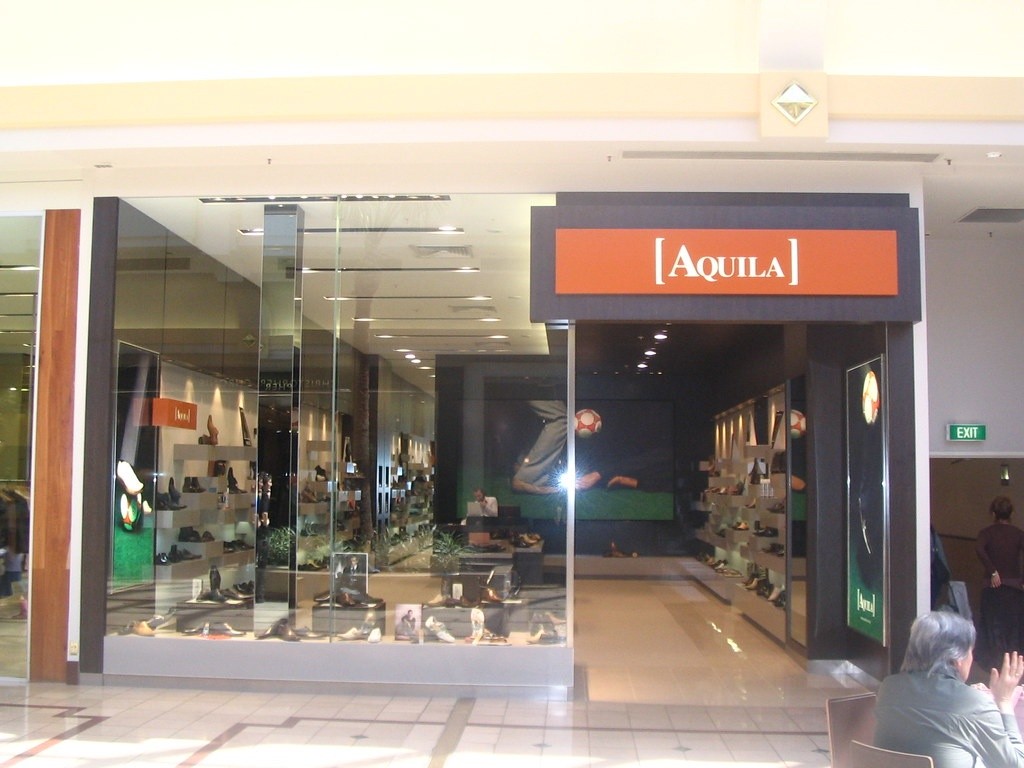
[946,579,972,622]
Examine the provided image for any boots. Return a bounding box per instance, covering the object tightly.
[12,599,28,620]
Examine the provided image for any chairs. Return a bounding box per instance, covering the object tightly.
[825,693,935,768]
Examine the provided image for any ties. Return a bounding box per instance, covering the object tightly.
[351,567,355,575]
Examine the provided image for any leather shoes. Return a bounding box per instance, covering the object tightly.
[474,527,541,553]
[208,414,218,445]
[228,467,238,491]
[429,586,504,608]
[157,525,255,567]
[156,476,206,510]
[254,617,381,643]
[313,587,383,608]
[695,454,787,611]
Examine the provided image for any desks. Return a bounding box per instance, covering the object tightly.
[457,540,546,585]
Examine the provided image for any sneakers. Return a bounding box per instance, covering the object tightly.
[131,620,155,636]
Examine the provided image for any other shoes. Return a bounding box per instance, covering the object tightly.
[184,621,243,636]
[197,565,254,602]
[391,471,435,546]
[301,463,366,553]
[603,549,626,558]
[426,615,567,645]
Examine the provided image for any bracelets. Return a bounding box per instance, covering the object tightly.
[992,571,999,576]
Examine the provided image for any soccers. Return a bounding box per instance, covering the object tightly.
[789,410,807,440]
[861,371,881,425]
[119,492,141,531]
[573,408,603,439]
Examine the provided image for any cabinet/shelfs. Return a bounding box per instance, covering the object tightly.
[152,441,434,605]
[693,444,806,647]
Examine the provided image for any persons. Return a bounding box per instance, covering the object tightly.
[0,545,28,620]
[973,497,1024,674]
[401,610,415,630]
[256,472,272,569]
[872,610,1024,768]
[931,526,951,609]
[473,488,498,517]
[511,400,668,495]
[343,556,366,592]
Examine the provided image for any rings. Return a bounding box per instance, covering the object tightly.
[1016,674,1020,676]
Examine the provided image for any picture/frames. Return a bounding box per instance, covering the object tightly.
[394,604,422,642]
[330,551,368,603]
